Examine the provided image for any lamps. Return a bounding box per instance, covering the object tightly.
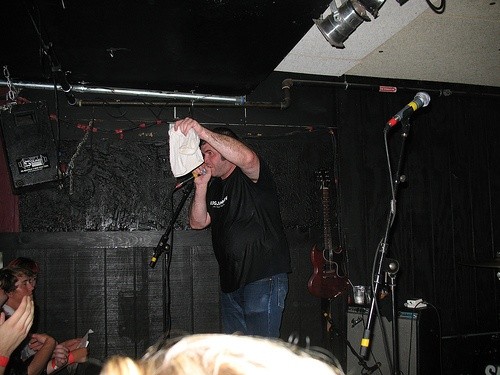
[312,0,408,47]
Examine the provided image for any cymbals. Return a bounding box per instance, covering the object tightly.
[462,256,499,269]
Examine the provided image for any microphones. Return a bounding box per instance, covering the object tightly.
[366,362,381,375]
[387,92,430,129]
[175,168,207,189]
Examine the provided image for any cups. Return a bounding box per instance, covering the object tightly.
[353,285,365,305]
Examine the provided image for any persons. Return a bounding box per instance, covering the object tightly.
[174,117,293,339]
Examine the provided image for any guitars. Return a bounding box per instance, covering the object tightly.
[303,167,366,313]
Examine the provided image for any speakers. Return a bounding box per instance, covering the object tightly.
[0,100,63,189]
[347,301,441,375]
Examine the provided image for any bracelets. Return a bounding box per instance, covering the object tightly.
[0,356,9,367]
[68,352,74,363]
[52,359,56,368]
[0,257,89,375]
